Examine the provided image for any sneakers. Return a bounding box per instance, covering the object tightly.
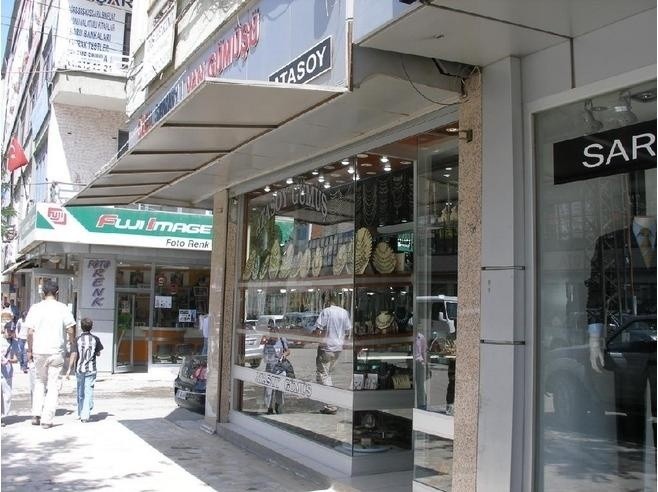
[267,403,280,415]
[32,416,52,428]
[81,419,87,423]
[319,407,337,415]
[1,418,6,427]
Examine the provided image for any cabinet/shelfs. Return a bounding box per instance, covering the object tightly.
[235,271,413,465]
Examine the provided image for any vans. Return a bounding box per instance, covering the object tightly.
[414,293,458,364]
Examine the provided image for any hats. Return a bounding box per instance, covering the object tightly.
[4,321,16,332]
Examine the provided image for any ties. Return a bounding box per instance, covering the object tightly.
[639,228,654,269]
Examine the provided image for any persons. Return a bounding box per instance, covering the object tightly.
[586,217,657,492]
[25,281,77,429]
[261,326,291,415]
[1,299,28,427]
[65,318,104,423]
[312,290,351,413]
[202,313,209,355]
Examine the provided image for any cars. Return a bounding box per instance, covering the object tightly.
[242,311,323,369]
[172,352,206,416]
[536,315,656,436]
